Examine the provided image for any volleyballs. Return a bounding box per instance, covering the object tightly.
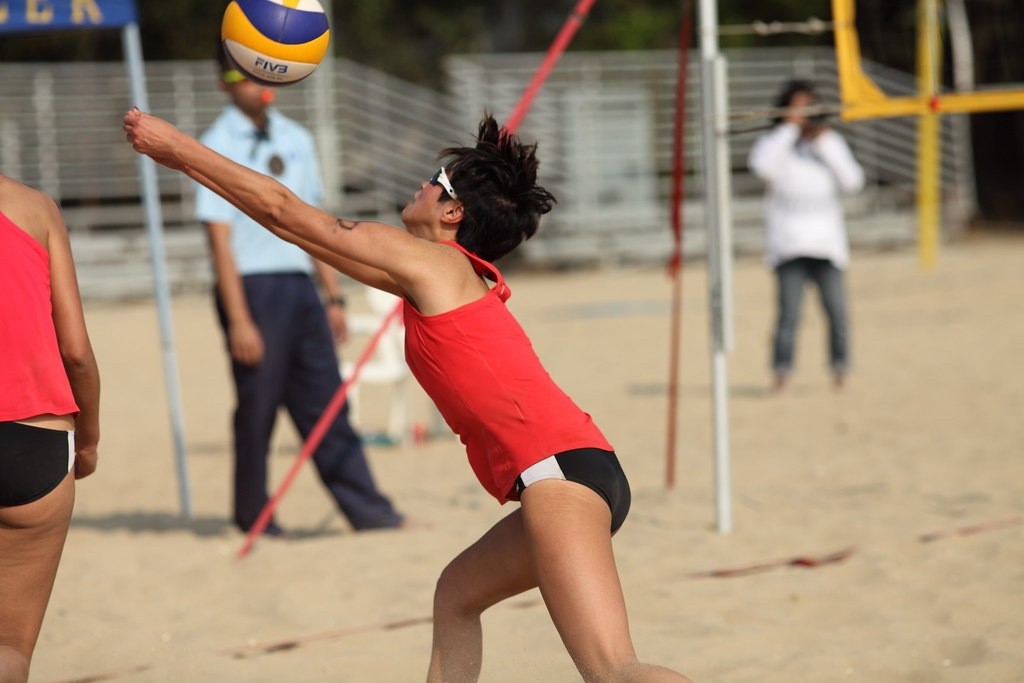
[220,0,333,87]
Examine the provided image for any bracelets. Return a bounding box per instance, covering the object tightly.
[324,297,344,306]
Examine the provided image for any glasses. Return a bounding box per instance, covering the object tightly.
[429,169,473,223]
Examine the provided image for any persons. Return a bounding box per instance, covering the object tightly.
[123,106,690,683]
[745,79,864,393]
[0,171,100,683]
[197,40,406,537]
